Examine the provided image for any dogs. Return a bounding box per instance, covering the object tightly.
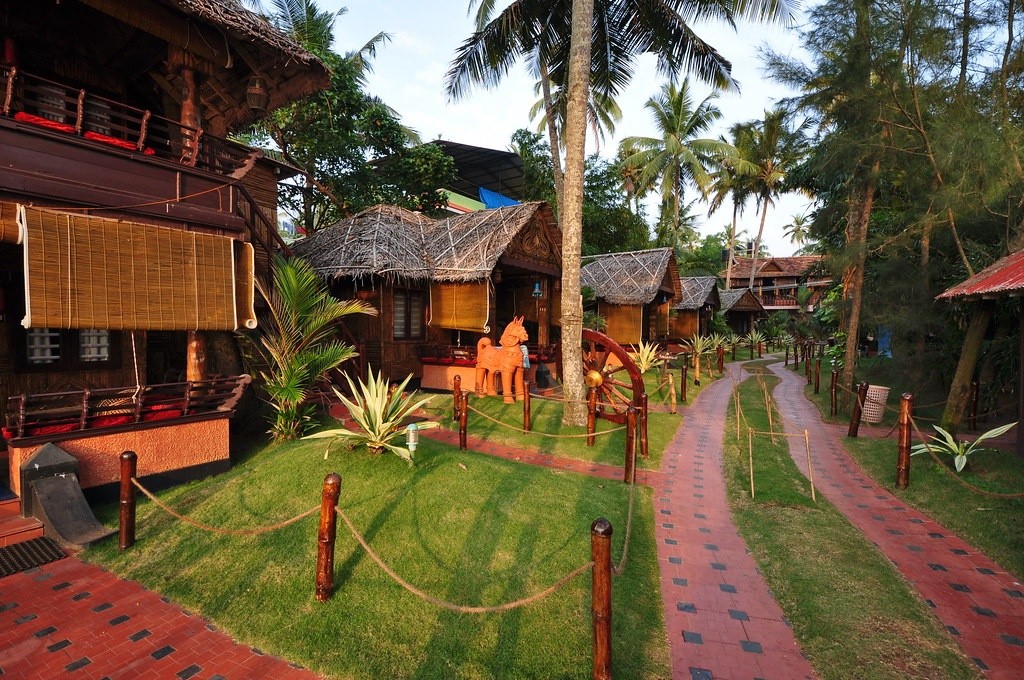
[474,315,529,404]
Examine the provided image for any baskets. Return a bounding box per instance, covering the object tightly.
[856,383,892,423]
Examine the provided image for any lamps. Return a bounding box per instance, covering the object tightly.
[405,423,419,445]
[531,274,542,298]
[662,292,667,303]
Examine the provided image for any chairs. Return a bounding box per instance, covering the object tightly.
[94,387,153,416]
